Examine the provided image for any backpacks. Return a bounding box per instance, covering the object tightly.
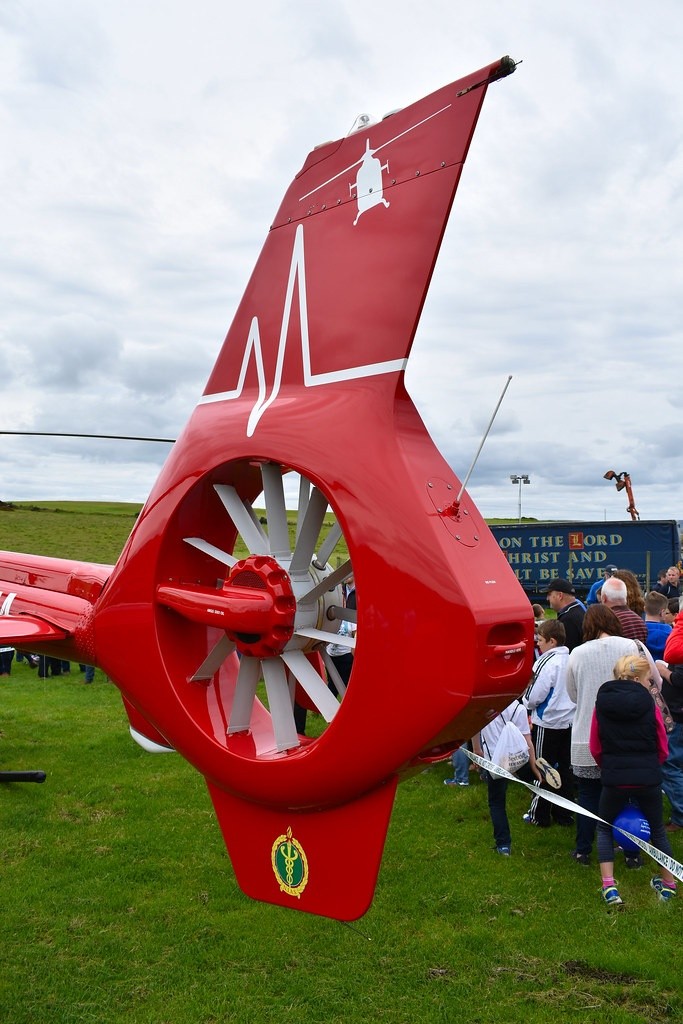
[483,704,530,778]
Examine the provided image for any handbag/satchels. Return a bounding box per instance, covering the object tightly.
[635,637,675,733]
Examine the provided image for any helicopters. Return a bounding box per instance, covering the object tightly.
[0,55,537,922]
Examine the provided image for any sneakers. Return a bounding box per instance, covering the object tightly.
[650,876,678,902]
[573,851,590,866]
[524,812,531,821]
[496,845,509,857]
[599,880,623,904]
[625,855,641,871]
[445,777,468,785]
[535,757,561,789]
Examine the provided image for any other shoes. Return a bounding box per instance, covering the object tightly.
[665,820,683,833]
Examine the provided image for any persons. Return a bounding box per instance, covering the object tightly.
[565,603,662,871]
[0,645,95,684]
[589,654,676,906]
[294,575,356,735]
[444,558,683,866]
[471,698,543,857]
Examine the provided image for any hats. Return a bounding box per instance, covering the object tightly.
[539,579,576,596]
[606,564,618,573]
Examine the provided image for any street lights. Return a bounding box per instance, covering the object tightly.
[510,474,530,523]
